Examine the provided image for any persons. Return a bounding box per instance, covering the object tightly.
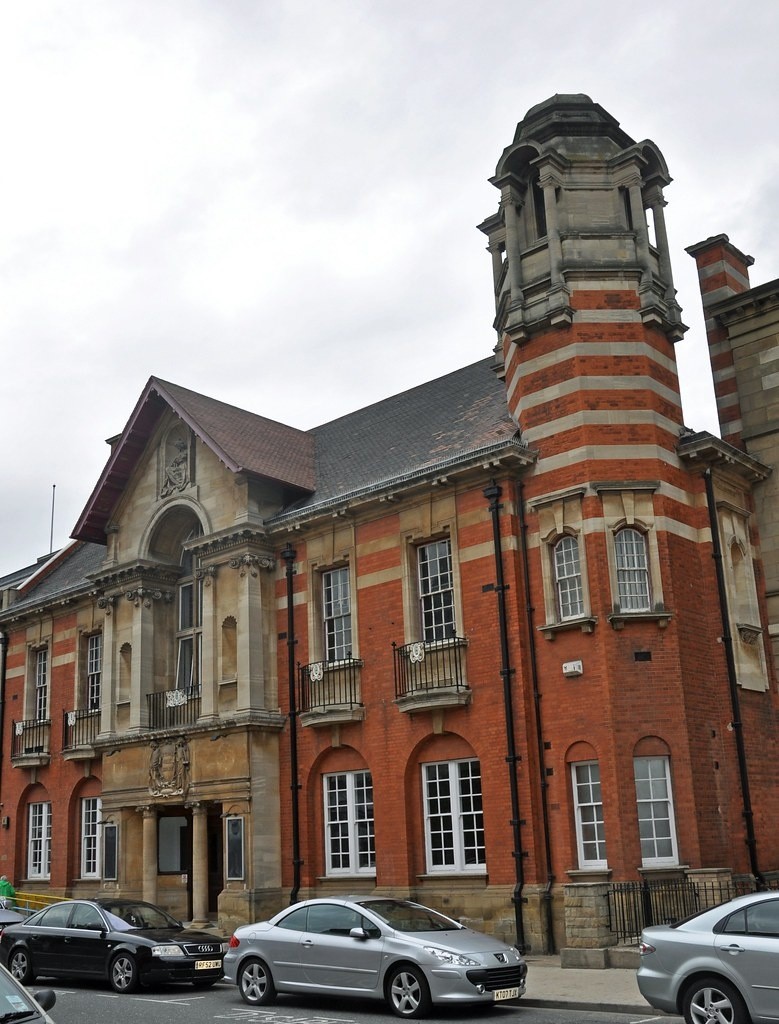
[0,875,16,912]
[174,737,190,788]
[150,741,164,782]
[165,439,189,495]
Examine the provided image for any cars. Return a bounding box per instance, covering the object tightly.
[0,957,59,1024]
[223,895,528,1018]
[637,890,779,1024]
[11,897,224,993]
